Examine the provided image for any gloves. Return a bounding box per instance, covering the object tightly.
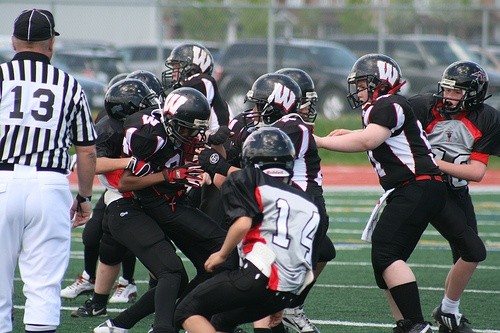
[127,155,162,196]
[163,162,203,189]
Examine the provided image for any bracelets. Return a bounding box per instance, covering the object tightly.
[74,192,92,213]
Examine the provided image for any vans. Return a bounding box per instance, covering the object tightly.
[0,37,500,123]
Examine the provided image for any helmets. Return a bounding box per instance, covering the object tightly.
[246,73,301,126]
[104,71,164,121]
[273,69,317,124]
[432,60,488,115]
[164,87,211,147]
[162,42,214,90]
[242,127,296,177]
[345,53,402,111]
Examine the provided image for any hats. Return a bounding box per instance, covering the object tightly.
[14,8,59,42]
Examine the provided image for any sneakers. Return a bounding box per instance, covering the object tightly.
[405,321,432,333]
[93,319,128,333]
[282,304,319,333]
[431,305,474,333]
[71,297,107,318]
[61,271,96,298]
[108,277,137,303]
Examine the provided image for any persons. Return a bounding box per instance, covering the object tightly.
[313,53,446,333]
[73,41,336,333]
[171,126,321,333]
[373,60,500,333]
[0,8,100,333]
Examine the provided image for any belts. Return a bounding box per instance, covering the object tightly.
[0,163,68,175]
